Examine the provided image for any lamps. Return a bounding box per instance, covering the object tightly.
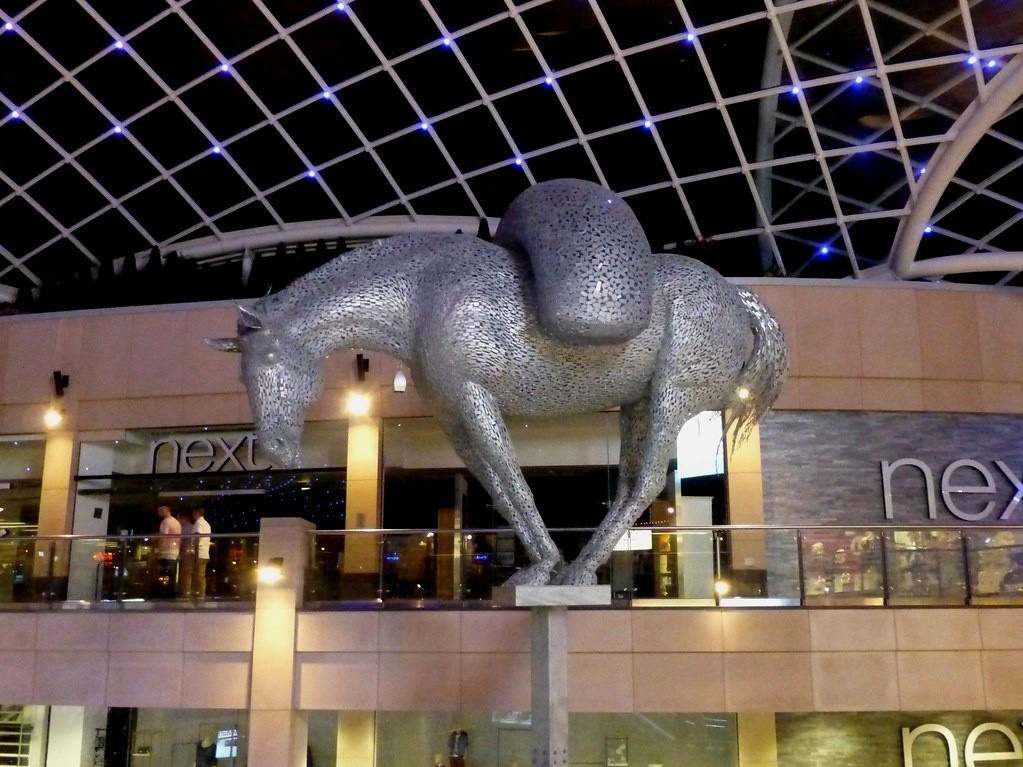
[393,360,407,393]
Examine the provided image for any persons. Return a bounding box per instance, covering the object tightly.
[194,736,218,767]
[448,727,468,767]
[176,507,212,602]
[434,753,446,767]
[156,505,182,602]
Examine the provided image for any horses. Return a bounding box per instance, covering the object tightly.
[200,227,792,587]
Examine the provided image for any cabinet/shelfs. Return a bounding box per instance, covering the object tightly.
[0,706,31,766]
